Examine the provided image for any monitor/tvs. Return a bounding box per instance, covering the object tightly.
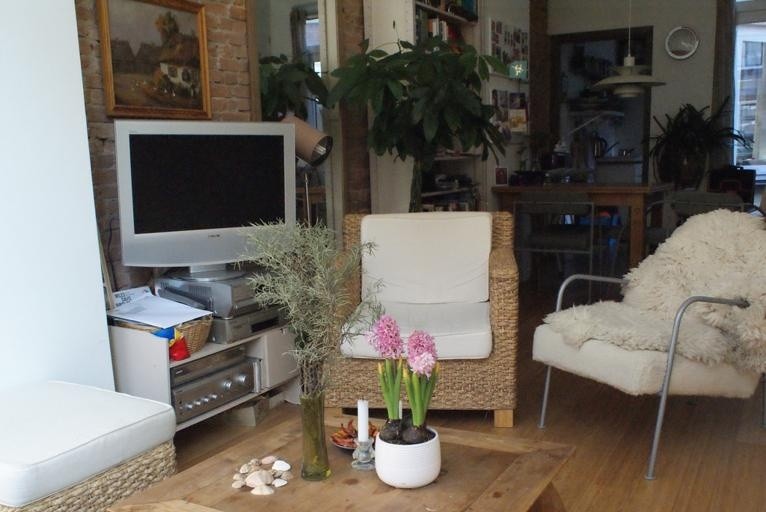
[113,118,295,280]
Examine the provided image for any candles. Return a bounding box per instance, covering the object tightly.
[356,395,370,441]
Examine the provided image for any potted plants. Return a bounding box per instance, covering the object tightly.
[639,97,750,183]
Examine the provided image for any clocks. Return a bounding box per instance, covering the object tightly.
[664,25,699,59]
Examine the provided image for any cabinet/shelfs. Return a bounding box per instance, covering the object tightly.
[364,0,488,211]
[105,296,305,433]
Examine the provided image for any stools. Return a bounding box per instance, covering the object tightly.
[1,378,177,511]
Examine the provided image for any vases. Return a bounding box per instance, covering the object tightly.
[375,426,441,489]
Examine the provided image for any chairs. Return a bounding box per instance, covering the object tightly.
[531,205,764,485]
[510,190,606,306]
[321,208,518,429]
[672,189,746,229]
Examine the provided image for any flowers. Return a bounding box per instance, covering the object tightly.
[368,316,443,444]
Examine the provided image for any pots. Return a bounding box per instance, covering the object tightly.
[618,149,635,160]
[592,136,607,157]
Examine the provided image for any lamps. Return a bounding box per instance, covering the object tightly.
[588,0,666,100]
[282,115,332,226]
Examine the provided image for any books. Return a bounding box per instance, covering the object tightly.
[415,0,462,54]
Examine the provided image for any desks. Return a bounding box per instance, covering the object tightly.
[107,413,581,512]
[295,185,327,224]
[495,181,674,295]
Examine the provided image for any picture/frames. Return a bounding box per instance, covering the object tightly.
[95,0,214,121]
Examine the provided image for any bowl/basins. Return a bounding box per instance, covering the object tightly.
[513,168,577,186]
